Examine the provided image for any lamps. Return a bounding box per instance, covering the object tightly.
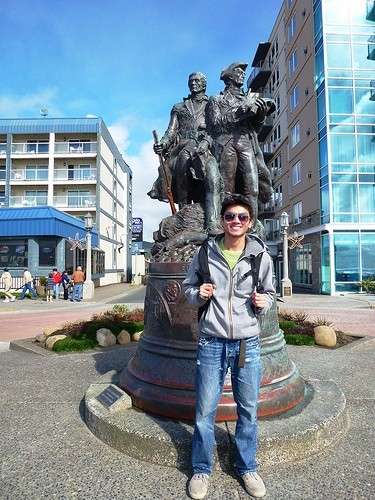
[62,187,65,192]
[63,162,66,166]
[63,136,66,141]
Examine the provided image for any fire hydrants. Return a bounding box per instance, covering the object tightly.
[131,279,136,286]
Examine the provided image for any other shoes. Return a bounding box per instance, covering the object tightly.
[3,300,9,303]
[11,296,16,301]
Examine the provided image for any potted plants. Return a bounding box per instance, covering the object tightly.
[39,276,48,287]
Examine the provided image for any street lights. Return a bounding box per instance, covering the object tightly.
[83,211,95,301]
[280,211,293,300]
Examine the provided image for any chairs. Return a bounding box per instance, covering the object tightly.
[84,200,96,207]
[0,202,5,208]
[30,200,37,206]
[70,146,83,153]
[0,149,6,155]
[21,200,29,207]
[89,172,96,180]
[28,149,36,154]
[15,150,22,155]
[14,172,22,180]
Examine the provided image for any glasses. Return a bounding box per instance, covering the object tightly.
[225,214,249,222]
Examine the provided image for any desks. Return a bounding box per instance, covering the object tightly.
[71,148,82,153]
[83,176,90,180]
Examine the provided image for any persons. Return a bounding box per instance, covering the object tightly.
[206,62,279,231]
[71,266,86,300]
[0,268,16,302]
[181,193,276,500]
[52,267,62,301]
[19,268,38,300]
[44,274,55,301]
[62,272,70,301]
[147,72,225,234]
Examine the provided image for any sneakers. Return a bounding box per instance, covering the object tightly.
[188,472,210,499]
[235,468,265,498]
